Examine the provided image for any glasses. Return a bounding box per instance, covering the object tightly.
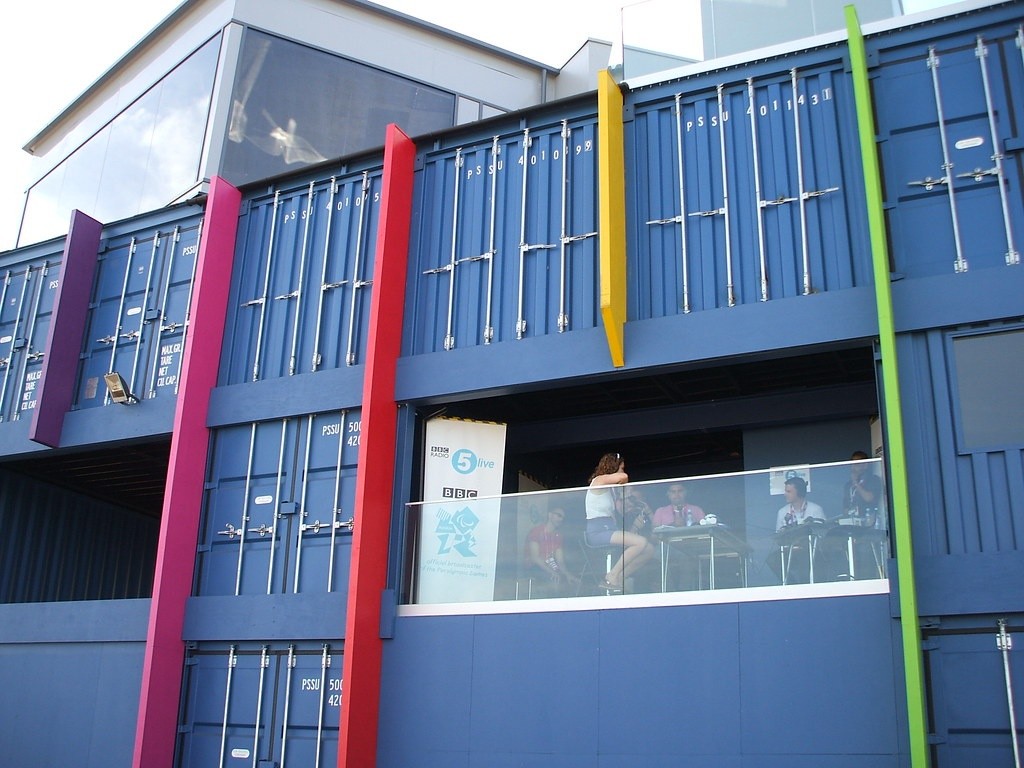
[553,513,565,519]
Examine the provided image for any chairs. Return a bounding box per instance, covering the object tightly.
[511,505,888,598]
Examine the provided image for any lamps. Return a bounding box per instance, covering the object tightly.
[104,371,141,407]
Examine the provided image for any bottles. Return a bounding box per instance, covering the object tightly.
[865,508,873,527]
[873,508,881,530]
[687,507,693,527]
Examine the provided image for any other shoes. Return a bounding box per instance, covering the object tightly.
[599,584,620,590]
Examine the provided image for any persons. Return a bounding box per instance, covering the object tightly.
[524,503,578,598]
[825,451,882,581]
[585,453,656,590]
[616,488,654,534]
[767,477,827,585]
[652,483,705,591]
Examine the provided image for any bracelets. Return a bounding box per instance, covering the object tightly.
[853,484,859,487]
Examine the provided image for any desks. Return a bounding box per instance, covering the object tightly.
[652,521,753,594]
[773,515,885,585]
